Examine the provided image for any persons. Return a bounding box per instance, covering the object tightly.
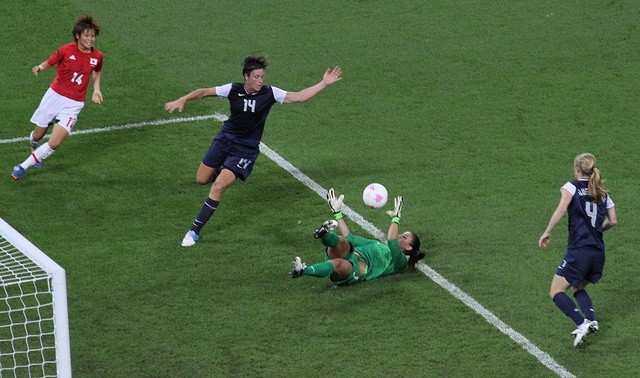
[537,153,618,347]
[10,15,104,181]
[164,56,342,247]
[289,188,425,287]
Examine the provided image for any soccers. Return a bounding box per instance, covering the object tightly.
[363,183,388,208]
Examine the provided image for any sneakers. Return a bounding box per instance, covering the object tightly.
[571,320,599,335]
[313,219,338,239]
[573,318,593,347]
[288,256,307,278]
[10,164,25,179]
[31,140,42,168]
[181,229,200,246]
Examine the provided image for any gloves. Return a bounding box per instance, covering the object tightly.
[326,188,344,220]
[386,195,404,225]
